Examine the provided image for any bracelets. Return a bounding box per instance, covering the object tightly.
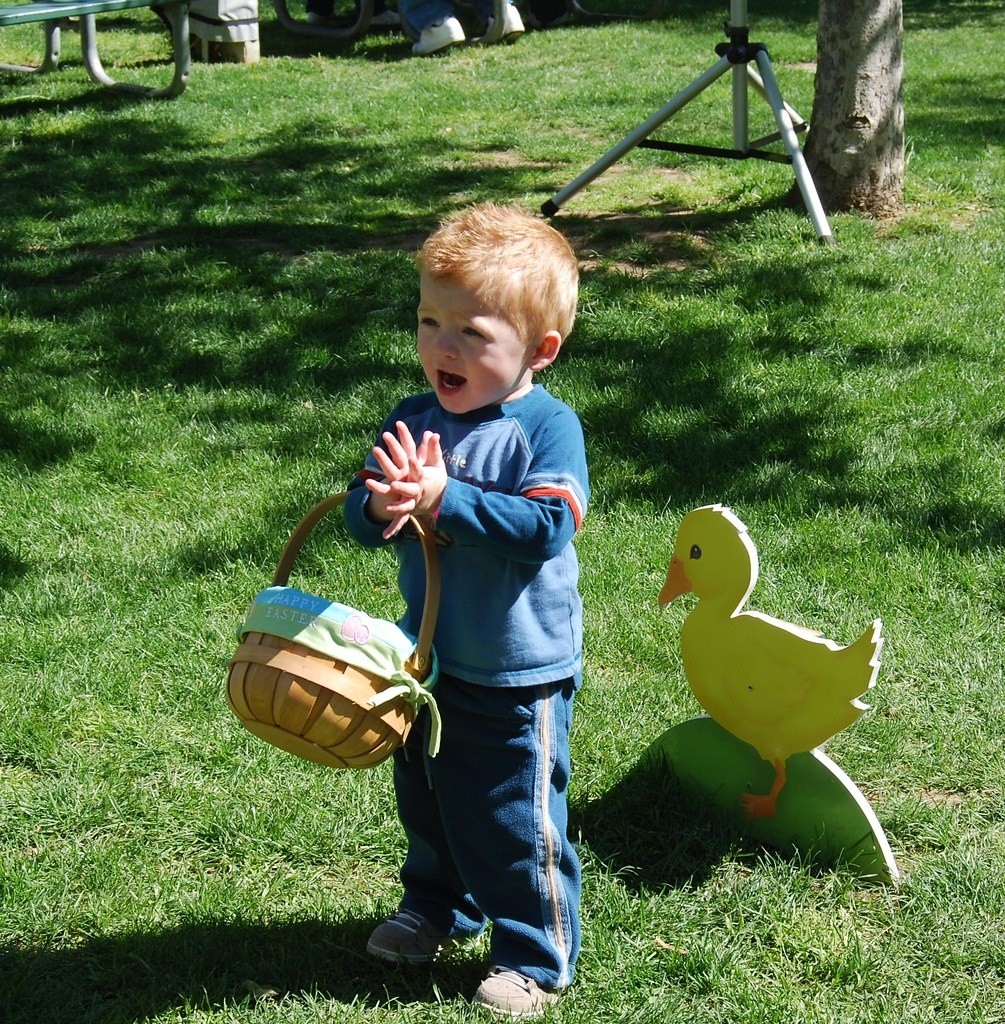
[433,503,443,521]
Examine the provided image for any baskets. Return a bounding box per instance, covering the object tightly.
[224,487,444,774]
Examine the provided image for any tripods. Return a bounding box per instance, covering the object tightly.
[540,0,837,248]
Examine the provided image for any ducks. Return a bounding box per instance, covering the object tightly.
[657,502,886,821]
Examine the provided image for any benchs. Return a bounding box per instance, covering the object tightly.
[0,0,373,102]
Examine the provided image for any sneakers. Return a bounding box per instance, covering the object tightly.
[367,906,476,962]
[468,966,565,1022]
[481,6,526,47]
[410,16,466,55]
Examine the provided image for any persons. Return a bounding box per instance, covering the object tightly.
[343,200,590,1023]
[304,0,524,54]
